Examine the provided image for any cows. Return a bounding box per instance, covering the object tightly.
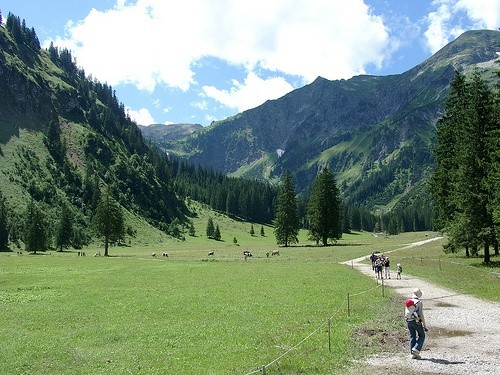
[266,250,280,257]
[243,251,253,258]
[207,250,214,256]
[151,252,157,257]
[162,252,169,258]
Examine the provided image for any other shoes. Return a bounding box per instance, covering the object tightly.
[411,351,421,358]
[411,355,416,359]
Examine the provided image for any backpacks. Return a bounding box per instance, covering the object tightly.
[399,266,402,272]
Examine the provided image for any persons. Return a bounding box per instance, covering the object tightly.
[381,255,385,273]
[375,256,384,279]
[383,257,391,279]
[396,263,401,280]
[405,287,428,359]
[405,299,420,322]
[370,252,378,271]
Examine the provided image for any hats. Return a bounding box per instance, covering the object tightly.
[397,264,400,266]
[413,288,422,298]
